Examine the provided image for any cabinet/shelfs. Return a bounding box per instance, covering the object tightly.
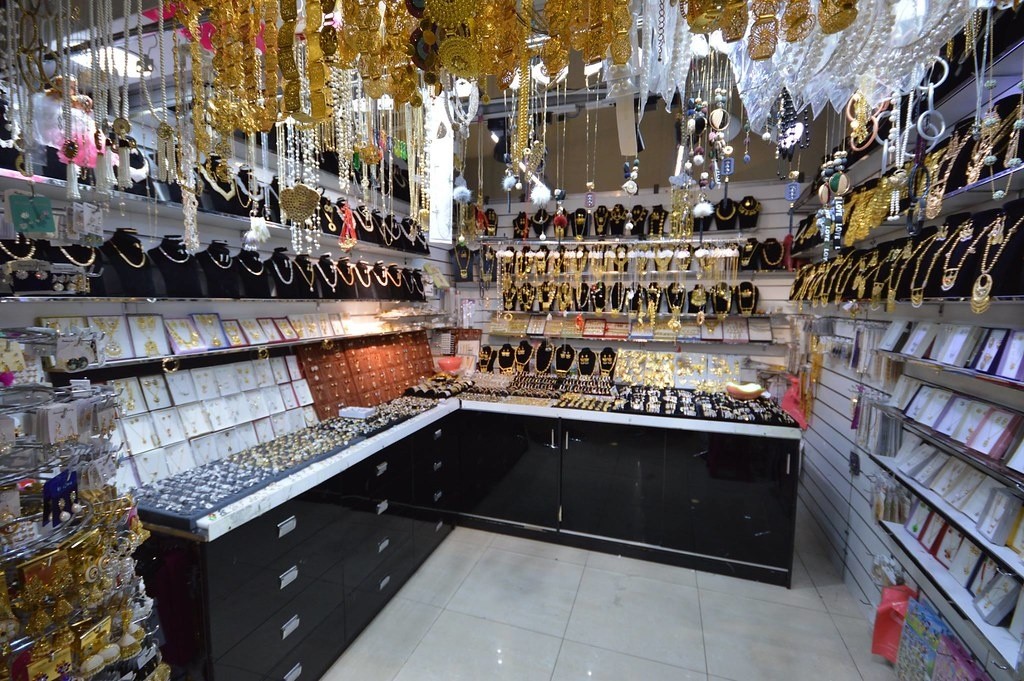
[477,227,796,347]
[131,397,460,681]
[455,395,802,591]
[869,347,1024,681]
[786,39,1024,307]
[0,167,430,312]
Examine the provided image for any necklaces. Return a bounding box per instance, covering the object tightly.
[158,244,190,263]
[111,234,147,266]
[315,260,337,294]
[239,255,264,275]
[0,0,1024,376]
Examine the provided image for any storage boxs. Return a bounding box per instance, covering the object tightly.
[917,511,948,554]
[936,324,984,367]
[930,456,969,496]
[909,451,951,489]
[976,487,1023,547]
[995,328,1024,382]
[959,475,1008,523]
[896,443,939,477]
[942,465,987,511]
[1006,505,1024,554]
[33,296,792,493]
[969,328,1011,376]
[973,569,1022,626]
[903,498,930,538]
[876,317,956,360]
[889,375,1024,477]
[966,553,1002,598]
[947,535,986,588]
[934,525,966,570]
